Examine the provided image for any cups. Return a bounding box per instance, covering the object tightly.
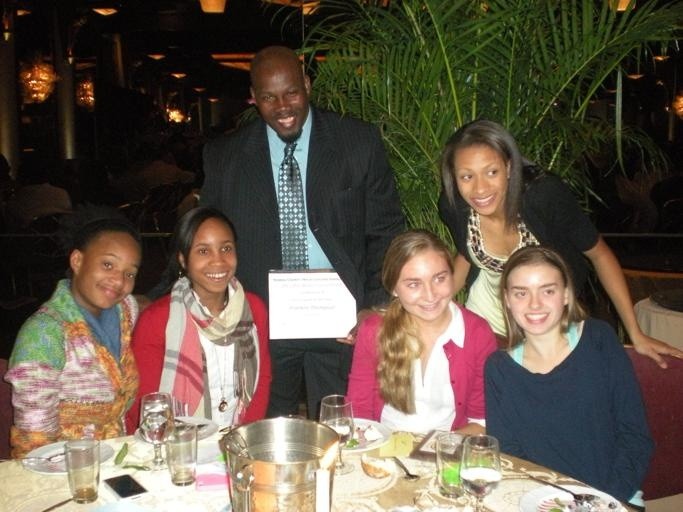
[63,438,101,506]
[163,422,199,487]
[433,431,464,500]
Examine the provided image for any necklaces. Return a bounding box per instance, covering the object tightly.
[192,282,234,412]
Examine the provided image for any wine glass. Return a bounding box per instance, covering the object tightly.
[138,391,175,472]
[317,395,353,476]
[458,434,502,512]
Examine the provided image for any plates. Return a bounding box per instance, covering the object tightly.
[518,485,626,512]
[386,458,436,481]
[173,416,217,442]
[21,439,116,475]
[345,456,399,500]
[320,416,390,451]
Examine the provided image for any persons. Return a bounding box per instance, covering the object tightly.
[1,92,205,375]
[125,207,269,437]
[1,219,155,463]
[436,117,683,371]
[339,231,498,439]
[485,244,658,512]
[197,45,410,419]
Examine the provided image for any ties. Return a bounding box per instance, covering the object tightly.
[278,143,308,269]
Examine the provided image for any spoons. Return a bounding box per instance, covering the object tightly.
[0,451,66,463]
[528,474,601,512]
[390,457,419,485]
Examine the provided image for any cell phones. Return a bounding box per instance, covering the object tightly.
[103,474,149,502]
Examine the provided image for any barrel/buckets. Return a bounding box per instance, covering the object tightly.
[223,414,341,512]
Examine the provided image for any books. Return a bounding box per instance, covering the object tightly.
[266,267,361,340]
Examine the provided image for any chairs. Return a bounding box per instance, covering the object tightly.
[606,344,682,500]
